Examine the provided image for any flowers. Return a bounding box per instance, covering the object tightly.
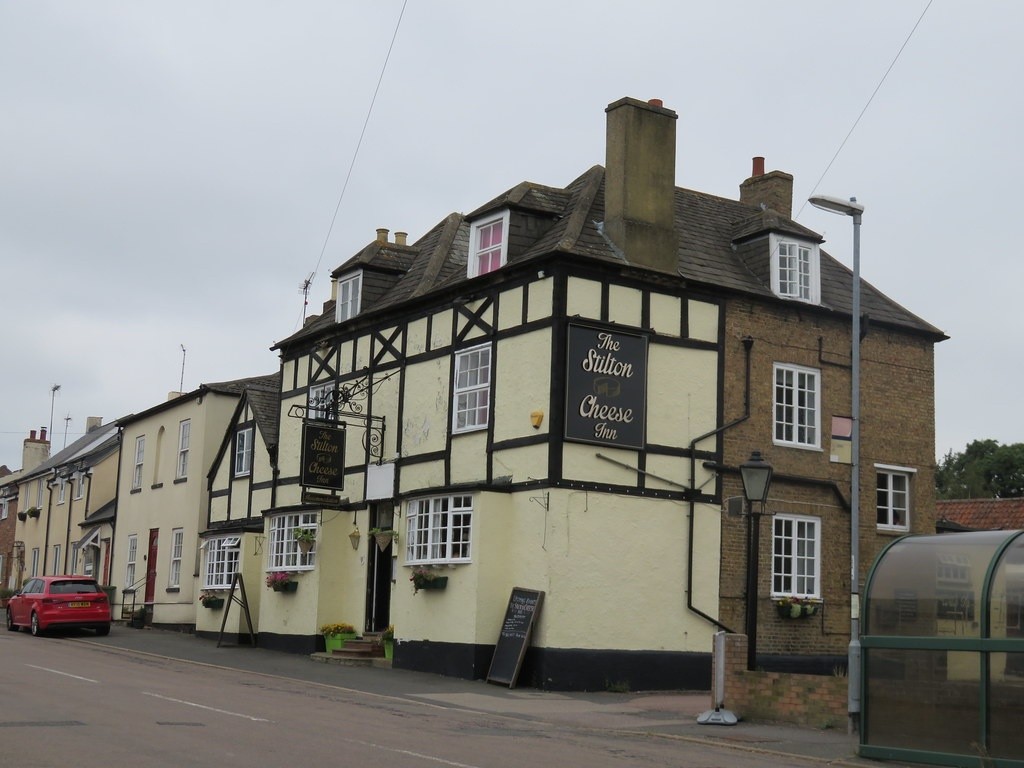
[319,623,356,639]
[265,572,290,590]
[409,568,439,596]
[199,595,217,606]
[377,625,394,646]
[778,597,818,618]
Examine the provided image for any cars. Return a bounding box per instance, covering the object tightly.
[6,576,109,636]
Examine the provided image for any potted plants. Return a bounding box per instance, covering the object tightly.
[292,529,314,556]
[0,589,13,608]
[368,528,398,552]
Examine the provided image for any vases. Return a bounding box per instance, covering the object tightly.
[325,633,356,652]
[414,576,448,590]
[273,581,298,593]
[204,599,224,609]
[790,604,815,617]
[384,641,393,659]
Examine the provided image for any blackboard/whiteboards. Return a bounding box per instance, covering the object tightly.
[487,586,546,685]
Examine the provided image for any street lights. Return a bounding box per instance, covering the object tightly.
[737,449,772,668]
[806,196,862,735]
